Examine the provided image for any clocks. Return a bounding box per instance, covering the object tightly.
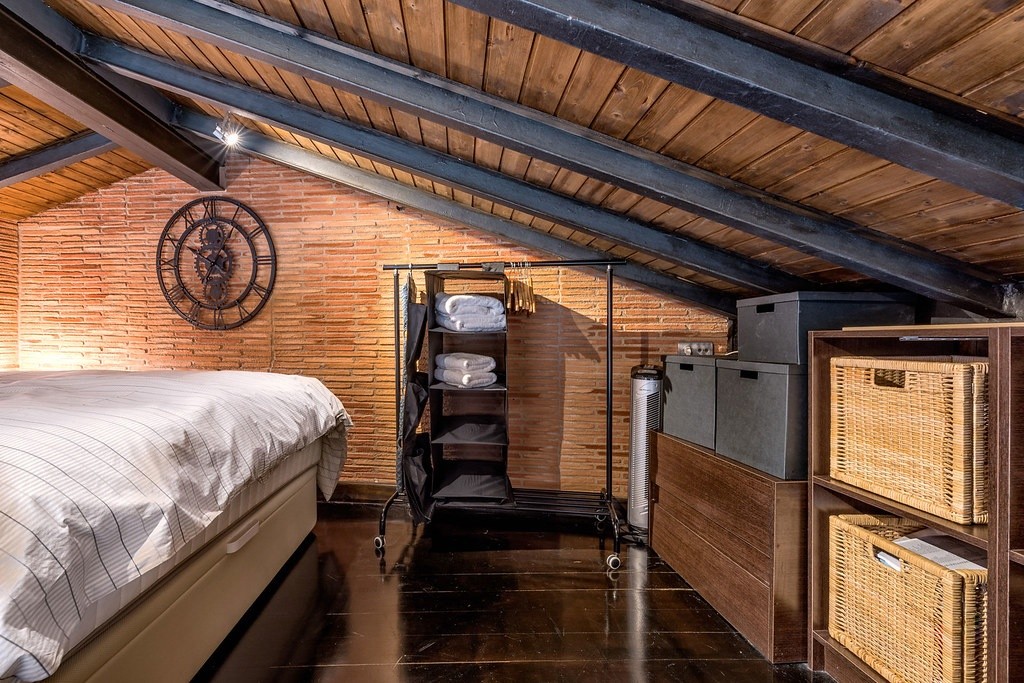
[156,197,277,331]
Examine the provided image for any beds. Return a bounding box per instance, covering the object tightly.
[0,370,352,683]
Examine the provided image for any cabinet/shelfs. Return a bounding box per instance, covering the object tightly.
[422,268,516,506]
[806,319,1024,683]
[647,428,806,665]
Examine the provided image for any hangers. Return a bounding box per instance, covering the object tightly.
[507,262,536,319]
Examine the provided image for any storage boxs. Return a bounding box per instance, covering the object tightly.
[663,293,929,481]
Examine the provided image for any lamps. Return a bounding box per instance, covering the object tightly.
[212,111,238,146]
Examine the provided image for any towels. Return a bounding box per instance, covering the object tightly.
[434,352,498,388]
[435,292,505,332]
